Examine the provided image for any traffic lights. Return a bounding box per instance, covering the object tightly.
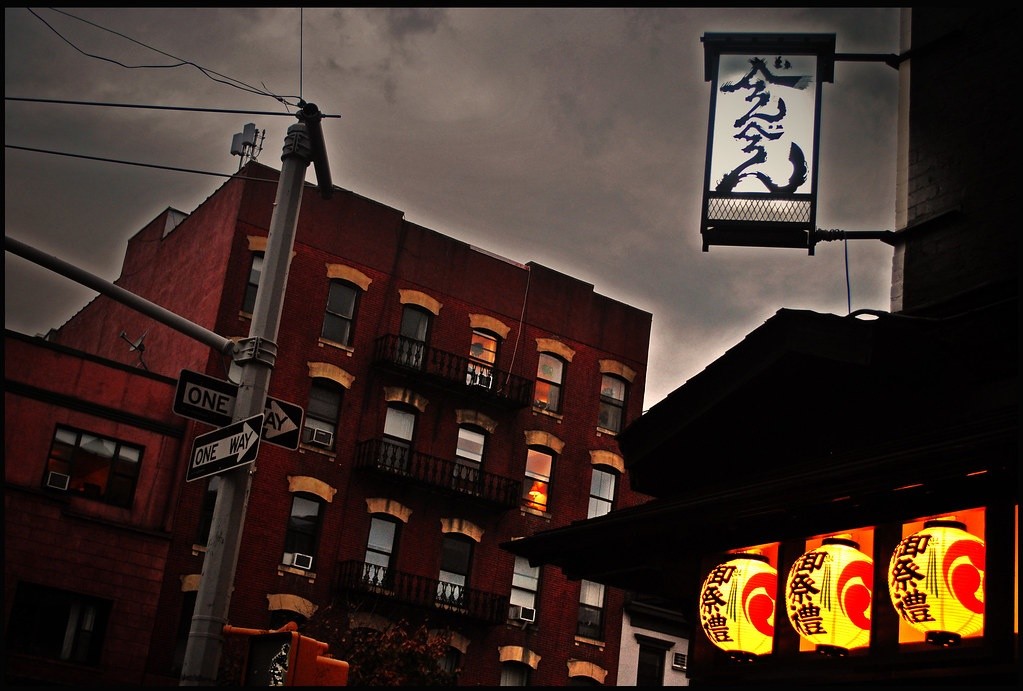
[286,631,349,686]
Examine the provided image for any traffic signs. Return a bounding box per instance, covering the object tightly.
[186,413,265,483]
[172,368,304,452]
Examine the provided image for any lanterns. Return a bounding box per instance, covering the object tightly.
[888,521,986,645]
[786,537,873,656]
[699,553,778,661]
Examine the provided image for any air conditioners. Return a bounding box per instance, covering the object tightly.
[515,606,537,623]
[46,471,70,490]
[309,427,333,447]
[291,552,313,570]
[469,373,493,388]
